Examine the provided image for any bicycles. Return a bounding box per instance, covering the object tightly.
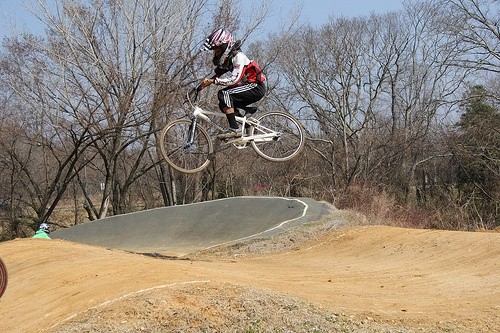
[159,79,305,174]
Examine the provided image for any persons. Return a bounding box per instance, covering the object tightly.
[186,29,268,142]
[31,223,52,239]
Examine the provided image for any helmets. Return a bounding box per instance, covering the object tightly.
[39,223,50,234]
[203,29,235,68]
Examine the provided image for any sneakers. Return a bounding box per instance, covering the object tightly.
[218,128,242,139]
